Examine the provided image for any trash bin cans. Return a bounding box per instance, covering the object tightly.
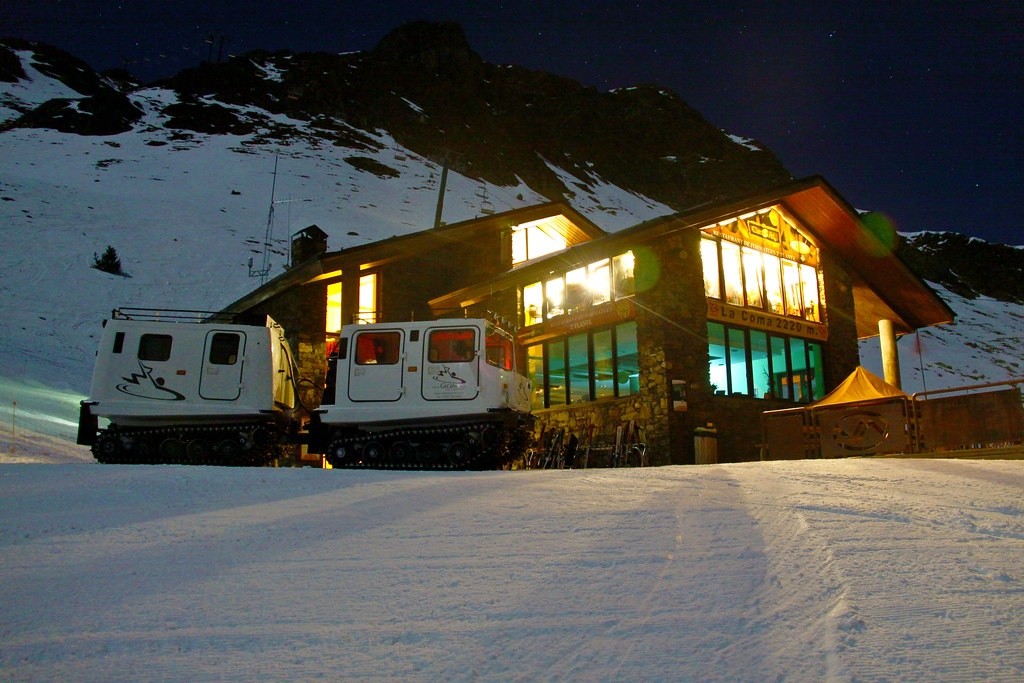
[693,426,718,465]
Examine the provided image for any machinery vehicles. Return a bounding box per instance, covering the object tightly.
[72,304,543,473]
